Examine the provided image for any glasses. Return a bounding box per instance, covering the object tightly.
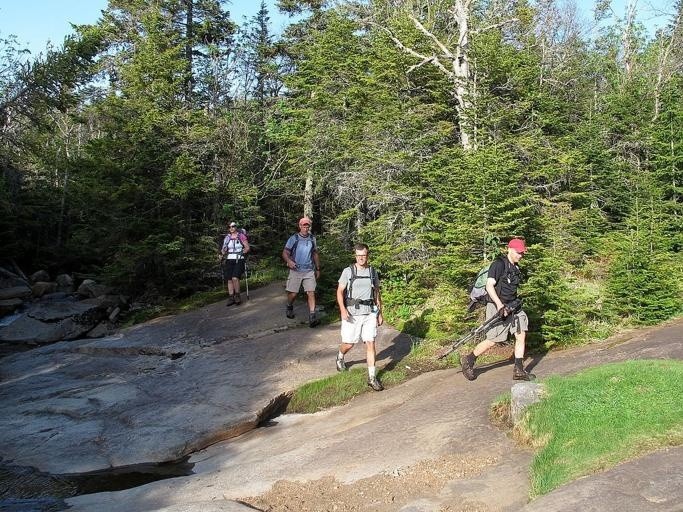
[228,225,235,228]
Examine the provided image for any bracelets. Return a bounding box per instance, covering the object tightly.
[316,266,321,271]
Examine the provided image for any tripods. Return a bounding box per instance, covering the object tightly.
[437,311,507,361]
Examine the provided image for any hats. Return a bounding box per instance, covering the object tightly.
[299,217,310,225]
[507,239,526,253]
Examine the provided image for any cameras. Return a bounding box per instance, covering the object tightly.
[509,296,525,314]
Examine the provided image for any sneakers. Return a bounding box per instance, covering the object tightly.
[458,355,476,381]
[286,305,294,319]
[511,365,535,381]
[335,353,347,373]
[309,315,320,328]
[226,299,234,306]
[234,295,241,303]
[367,376,382,391]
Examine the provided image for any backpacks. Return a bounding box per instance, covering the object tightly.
[469,257,510,306]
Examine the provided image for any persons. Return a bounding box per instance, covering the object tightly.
[459,239,537,381]
[281,217,322,327]
[219,221,250,306]
[335,243,384,392]
